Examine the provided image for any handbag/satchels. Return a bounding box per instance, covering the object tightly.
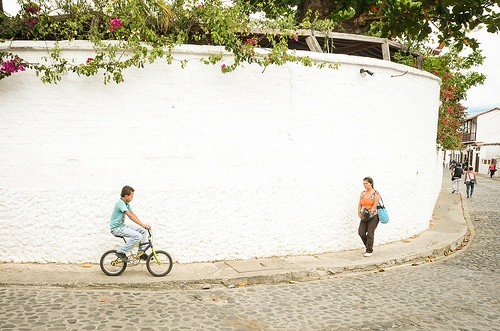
[451,176,455,181]
[377,197,389,224]
[471,179,476,183]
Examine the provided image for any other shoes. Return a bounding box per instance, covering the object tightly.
[452,189,456,193]
[467,195,473,198]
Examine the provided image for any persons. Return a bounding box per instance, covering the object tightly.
[110,186,154,261]
[358,177,380,256]
[449,158,496,198]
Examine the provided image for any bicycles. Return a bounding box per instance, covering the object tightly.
[100,229,173,278]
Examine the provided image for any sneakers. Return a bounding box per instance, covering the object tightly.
[137,252,154,260]
[115,251,132,262]
[364,248,374,256]
